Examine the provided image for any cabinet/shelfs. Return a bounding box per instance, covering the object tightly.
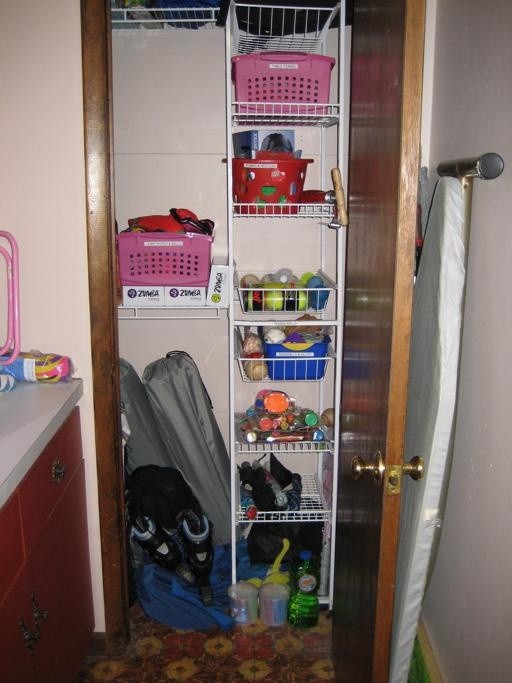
[0,376,96,683]
[223,0,348,609]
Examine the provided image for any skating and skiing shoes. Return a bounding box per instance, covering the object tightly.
[178,513,217,607]
[129,513,195,588]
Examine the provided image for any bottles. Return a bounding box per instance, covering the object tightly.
[258,583,288,626]
[228,583,258,625]
[288,550,320,629]
[274,267,292,286]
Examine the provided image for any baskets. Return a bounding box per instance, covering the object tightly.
[231,53,336,119]
[116,228,214,290]
[221,156,316,214]
